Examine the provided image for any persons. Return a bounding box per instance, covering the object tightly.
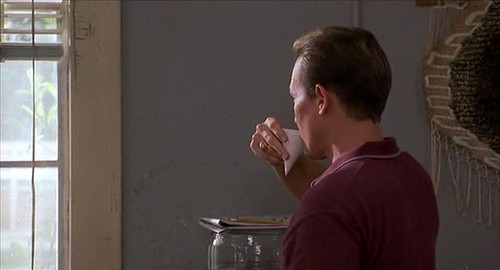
[250,25,440,270]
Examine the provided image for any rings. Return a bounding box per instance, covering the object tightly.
[259,140,269,151]
